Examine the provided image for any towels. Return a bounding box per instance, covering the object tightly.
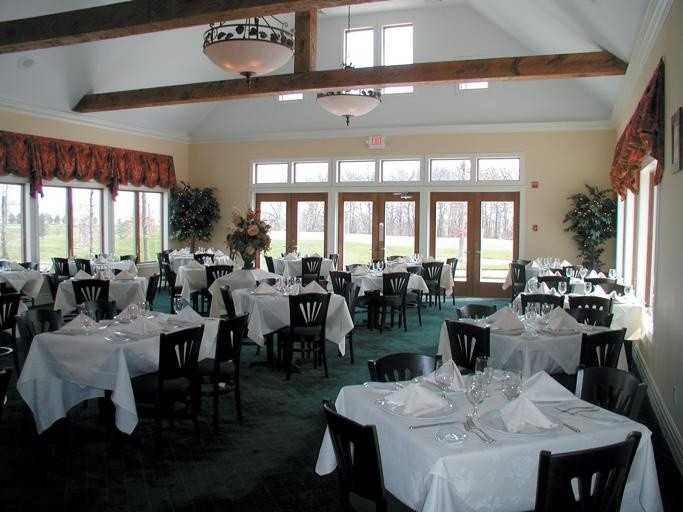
[594,285,605,296]
[295,284,308,295]
[561,260,571,266]
[423,256,435,263]
[521,371,577,401]
[206,249,213,253]
[179,248,186,254]
[215,250,224,256]
[545,270,554,276]
[609,291,618,300]
[549,306,577,331]
[311,253,321,258]
[393,266,407,273]
[285,254,297,260]
[424,360,465,392]
[589,270,597,278]
[62,312,99,331]
[10,261,25,271]
[75,270,91,280]
[126,316,161,334]
[171,250,180,255]
[387,383,448,416]
[116,270,133,280]
[487,309,522,331]
[304,281,327,293]
[353,266,370,276]
[178,306,204,323]
[95,271,109,280]
[395,257,404,264]
[528,261,538,268]
[500,395,557,433]
[188,260,202,268]
[255,283,272,293]
[383,267,392,273]
[116,303,144,321]
[597,272,606,278]
[96,256,105,264]
[540,282,549,293]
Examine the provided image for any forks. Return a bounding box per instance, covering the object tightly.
[467,418,496,444]
[567,410,601,417]
[464,422,490,445]
[555,406,596,412]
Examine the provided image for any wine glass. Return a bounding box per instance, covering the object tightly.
[379,262,385,273]
[468,375,487,424]
[140,302,150,321]
[529,281,538,294]
[567,268,573,278]
[509,303,520,319]
[275,275,302,296]
[542,304,555,331]
[580,269,588,280]
[130,306,139,332]
[476,358,494,398]
[82,311,94,336]
[584,283,592,297]
[374,264,379,279]
[558,282,567,296]
[174,298,187,321]
[502,370,522,403]
[526,303,539,337]
[437,361,455,400]
[415,254,424,265]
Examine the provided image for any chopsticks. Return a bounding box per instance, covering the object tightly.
[564,423,581,434]
[410,422,458,430]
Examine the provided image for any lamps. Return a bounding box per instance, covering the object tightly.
[314,4,380,125]
[202,14,297,86]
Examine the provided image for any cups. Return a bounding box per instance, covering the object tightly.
[625,286,634,295]
[609,269,616,279]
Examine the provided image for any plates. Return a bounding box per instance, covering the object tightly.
[542,327,579,335]
[364,382,407,394]
[484,326,527,335]
[433,429,466,446]
[376,397,458,421]
[479,400,564,437]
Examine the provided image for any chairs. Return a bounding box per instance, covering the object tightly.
[443,258,457,306]
[454,304,496,320]
[345,264,364,272]
[368,352,442,381]
[507,262,525,302]
[264,256,275,273]
[335,283,359,363]
[422,262,443,310]
[220,284,272,354]
[329,254,338,270]
[582,276,617,294]
[0,347,13,420]
[568,295,612,312]
[0,294,21,352]
[277,292,332,379]
[197,313,247,423]
[300,257,322,286]
[146,274,158,311]
[318,399,387,512]
[17,309,63,358]
[519,293,564,315]
[74,258,91,275]
[536,275,570,293]
[374,272,410,333]
[536,431,641,512]
[574,363,646,420]
[327,269,373,332]
[157,253,172,294]
[51,258,70,293]
[170,273,198,313]
[201,264,233,317]
[578,328,627,369]
[192,253,214,263]
[134,324,203,444]
[444,320,491,373]
[71,280,109,319]
